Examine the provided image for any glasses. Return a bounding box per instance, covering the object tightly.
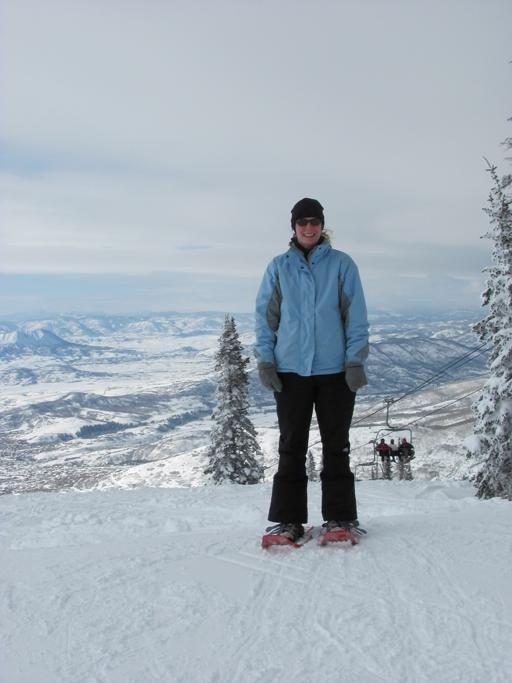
[293,217,322,226]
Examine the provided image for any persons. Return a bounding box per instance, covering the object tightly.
[397,438,416,460]
[376,439,391,461]
[388,439,399,461]
[256,196,372,546]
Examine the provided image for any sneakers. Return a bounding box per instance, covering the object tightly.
[327,519,350,533]
[274,520,305,542]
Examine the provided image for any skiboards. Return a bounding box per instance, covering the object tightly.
[391,458,403,464]
[261,522,362,549]
[403,455,415,464]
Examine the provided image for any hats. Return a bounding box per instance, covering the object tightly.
[290,197,324,227]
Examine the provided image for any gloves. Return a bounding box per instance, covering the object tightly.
[342,362,368,393]
[256,361,283,393]
